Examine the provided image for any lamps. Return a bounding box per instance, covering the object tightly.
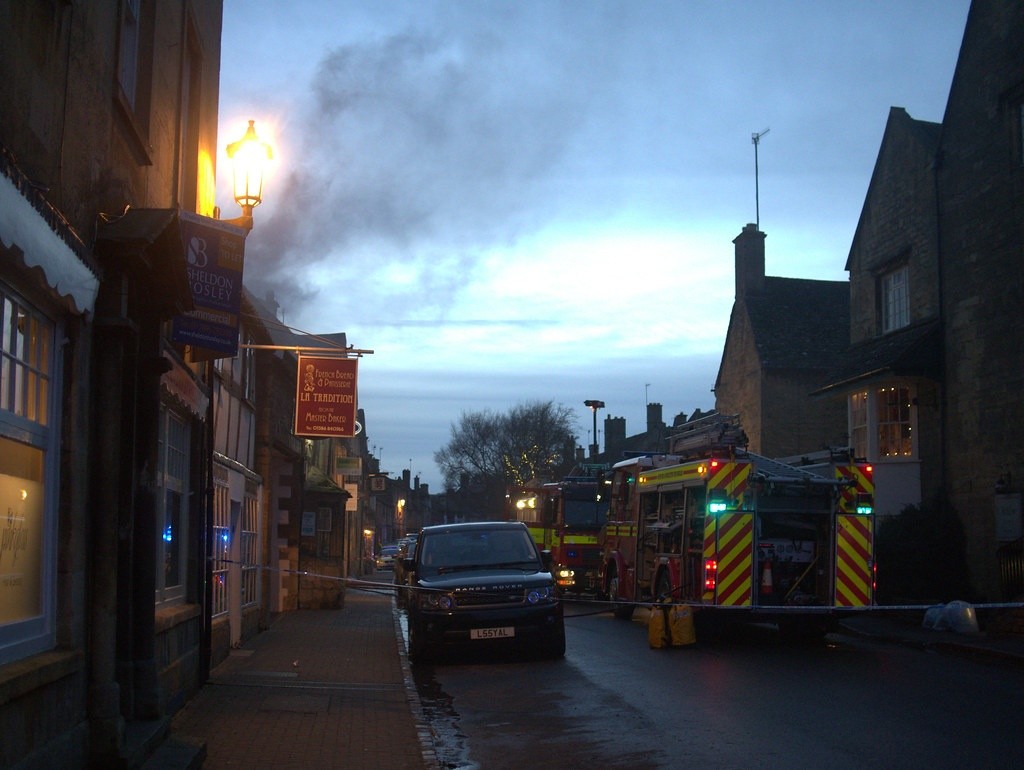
[215,117,274,226]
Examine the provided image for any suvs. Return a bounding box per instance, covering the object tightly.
[401,519,568,668]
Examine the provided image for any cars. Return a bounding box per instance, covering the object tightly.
[396,533,418,577]
[376,549,398,570]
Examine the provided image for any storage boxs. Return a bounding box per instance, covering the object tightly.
[758,538,816,562]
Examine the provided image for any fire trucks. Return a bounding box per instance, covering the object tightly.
[599,412,877,634]
[512,463,606,590]
